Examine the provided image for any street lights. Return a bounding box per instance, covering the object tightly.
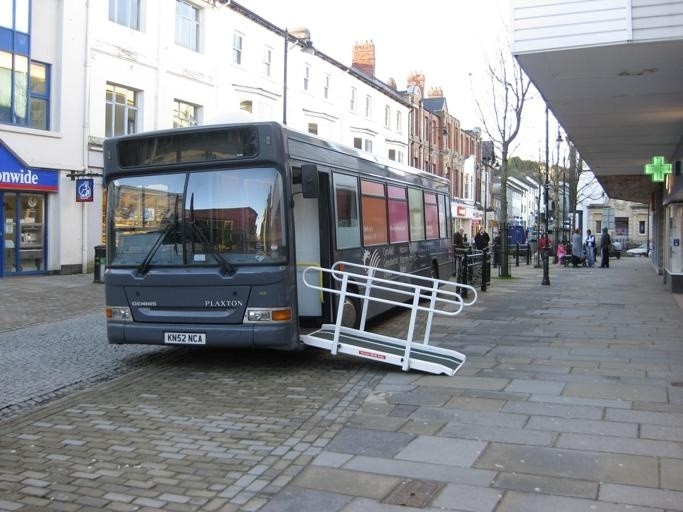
[283,27,315,124]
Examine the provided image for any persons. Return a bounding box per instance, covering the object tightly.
[613,239,622,257]
[540,232,552,260]
[571,229,582,268]
[585,229,596,268]
[474,226,490,250]
[556,240,567,266]
[493,231,502,268]
[454,227,468,248]
[598,227,611,268]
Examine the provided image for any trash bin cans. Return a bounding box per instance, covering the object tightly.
[94,245,106,282]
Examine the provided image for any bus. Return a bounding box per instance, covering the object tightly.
[103,121,477,377]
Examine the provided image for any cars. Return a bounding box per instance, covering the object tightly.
[627,243,653,257]
